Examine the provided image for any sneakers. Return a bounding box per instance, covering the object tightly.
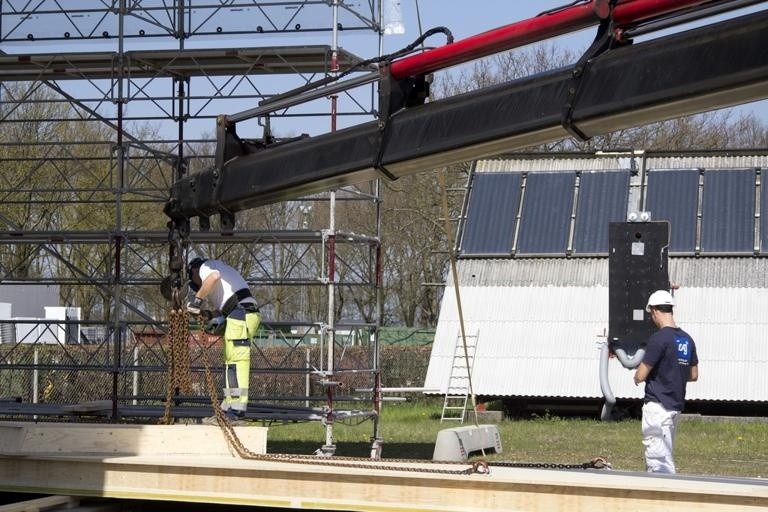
[202,411,245,427]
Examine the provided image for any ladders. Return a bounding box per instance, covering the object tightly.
[440,329,480,425]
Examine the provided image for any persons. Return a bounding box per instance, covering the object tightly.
[186,258,260,424]
[635,290,699,474]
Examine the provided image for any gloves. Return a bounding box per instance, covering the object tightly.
[199,310,212,323]
[187,298,203,316]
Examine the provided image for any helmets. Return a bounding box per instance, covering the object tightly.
[645,289,676,313]
[187,257,201,293]
